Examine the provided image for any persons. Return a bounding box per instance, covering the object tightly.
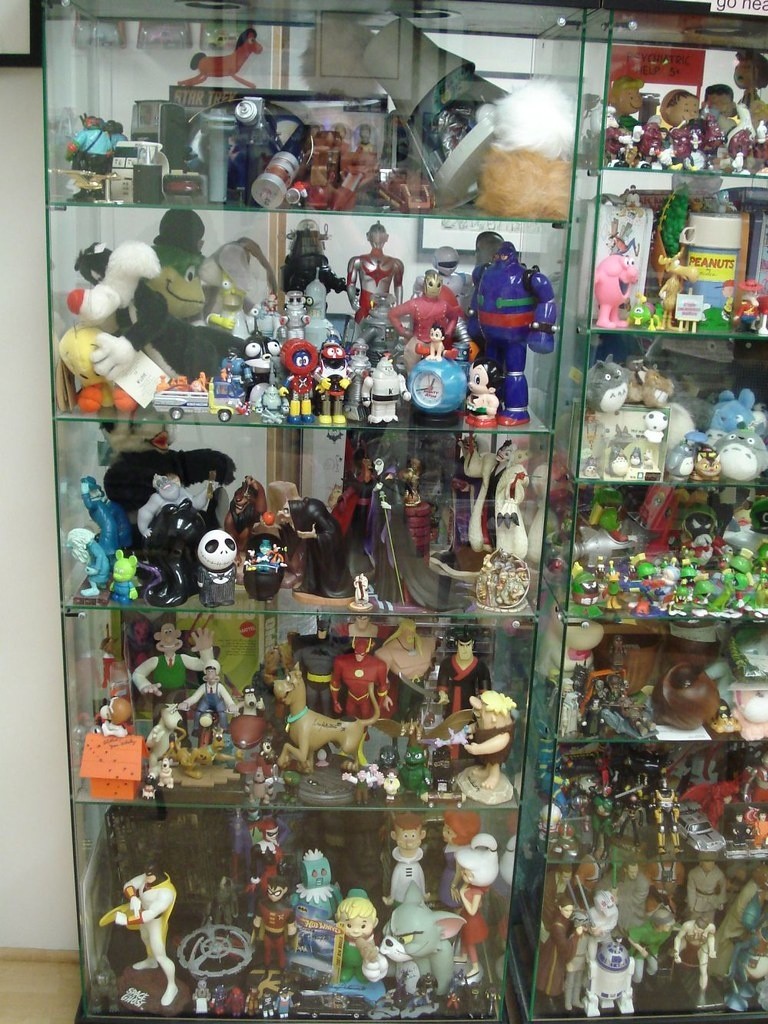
[223,448,472,612]
[732,280,762,333]
[285,809,502,1021]
[114,807,296,1019]
[64,117,130,198]
[530,743,768,857]
[456,434,529,560]
[679,503,720,571]
[153,216,412,425]
[554,674,660,738]
[132,618,239,762]
[538,852,768,1018]
[66,473,237,607]
[289,616,518,801]
[385,229,557,428]
[610,49,768,132]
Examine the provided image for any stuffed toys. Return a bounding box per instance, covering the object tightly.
[582,355,768,482]
[99,421,237,549]
[58,209,276,414]
[707,623,768,741]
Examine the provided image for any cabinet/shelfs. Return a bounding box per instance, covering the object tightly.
[48,1,592,1020]
[510,1,767,1024]
